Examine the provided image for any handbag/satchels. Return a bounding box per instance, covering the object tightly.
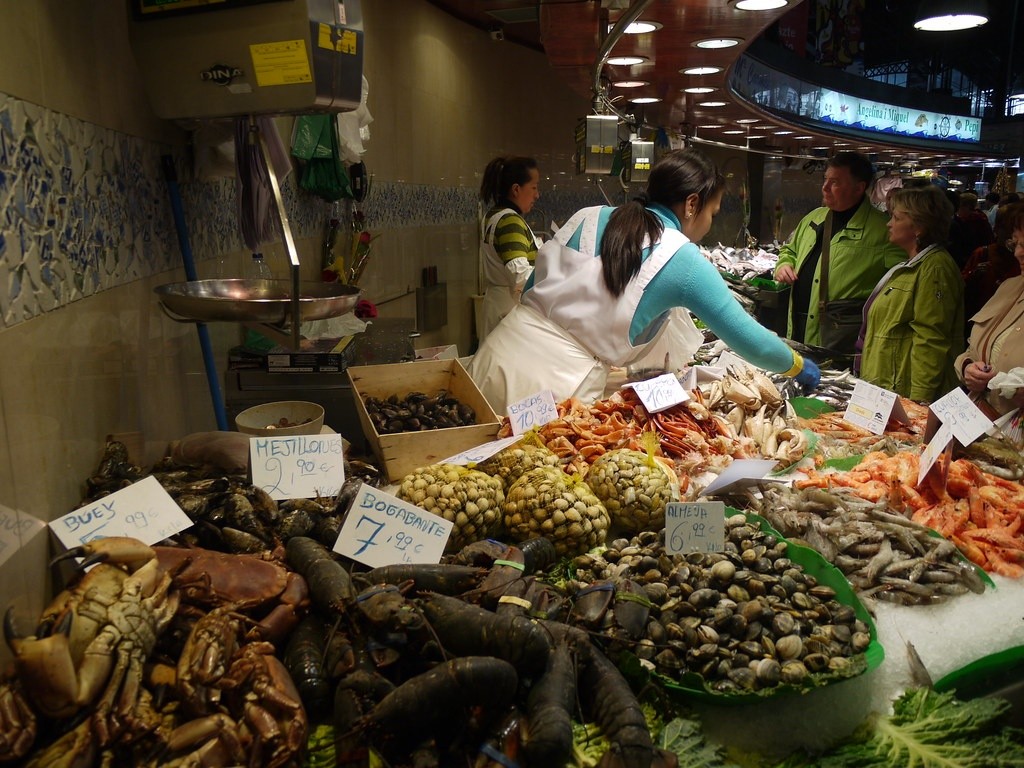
[818,300,866,368]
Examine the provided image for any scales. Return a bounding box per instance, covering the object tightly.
[125,0,366,353]
[575,113,655,182]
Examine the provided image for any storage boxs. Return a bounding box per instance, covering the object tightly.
[346,357,503,485]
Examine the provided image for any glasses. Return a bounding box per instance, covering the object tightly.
[1005,239,1024,252]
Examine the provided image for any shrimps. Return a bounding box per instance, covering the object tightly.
[728,394,1023,604]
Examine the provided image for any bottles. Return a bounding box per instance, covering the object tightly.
[249,253,273,279]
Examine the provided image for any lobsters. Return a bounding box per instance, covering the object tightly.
[286,537,678,767]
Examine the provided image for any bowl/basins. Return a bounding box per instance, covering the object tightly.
[234,401,325,437]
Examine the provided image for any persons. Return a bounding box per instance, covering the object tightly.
[478,155,541,347]
[852,182,968,408]
[948,187,1024,353]
[465,148,821,423]
[951,203,1024,418]
[771,152,908,373]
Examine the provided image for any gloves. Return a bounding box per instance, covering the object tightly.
[795,359,821,390]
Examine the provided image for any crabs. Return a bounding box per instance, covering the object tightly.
[488,385,757,503]
[0,535,307,768]
